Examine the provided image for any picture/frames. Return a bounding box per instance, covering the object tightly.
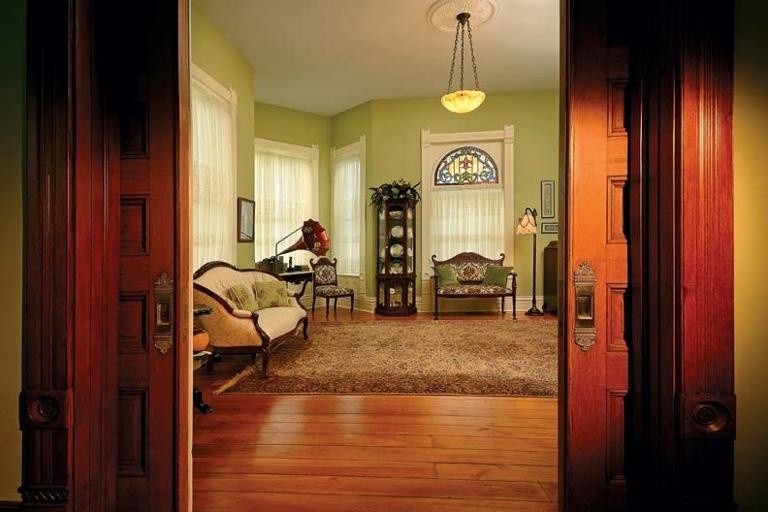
[238,197,255,243]
[541,223,560,234]
[541,179,555,218]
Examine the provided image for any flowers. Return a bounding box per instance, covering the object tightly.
[368,177,422,211]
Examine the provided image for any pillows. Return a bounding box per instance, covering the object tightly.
[229,284,259,314]
[253,281,287,310]
[431,265,460,288]
[481,263,513,288]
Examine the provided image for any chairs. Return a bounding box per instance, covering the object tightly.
[309,258,354,318]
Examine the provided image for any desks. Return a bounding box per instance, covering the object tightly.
[278,270,314,311]
[193,346,222,413]
[543,241,558,314]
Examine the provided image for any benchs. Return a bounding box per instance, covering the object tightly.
[431,252,519,321]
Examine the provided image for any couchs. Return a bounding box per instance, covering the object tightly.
[193,261,309,378]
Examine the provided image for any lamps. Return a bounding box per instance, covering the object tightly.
[514,208,545,316]
[441,13,487,115]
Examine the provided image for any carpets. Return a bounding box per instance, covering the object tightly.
[213,319,559,398]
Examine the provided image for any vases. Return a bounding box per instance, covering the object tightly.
[193,326,210,353]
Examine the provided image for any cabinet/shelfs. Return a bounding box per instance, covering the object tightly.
[376,198,418,316]
